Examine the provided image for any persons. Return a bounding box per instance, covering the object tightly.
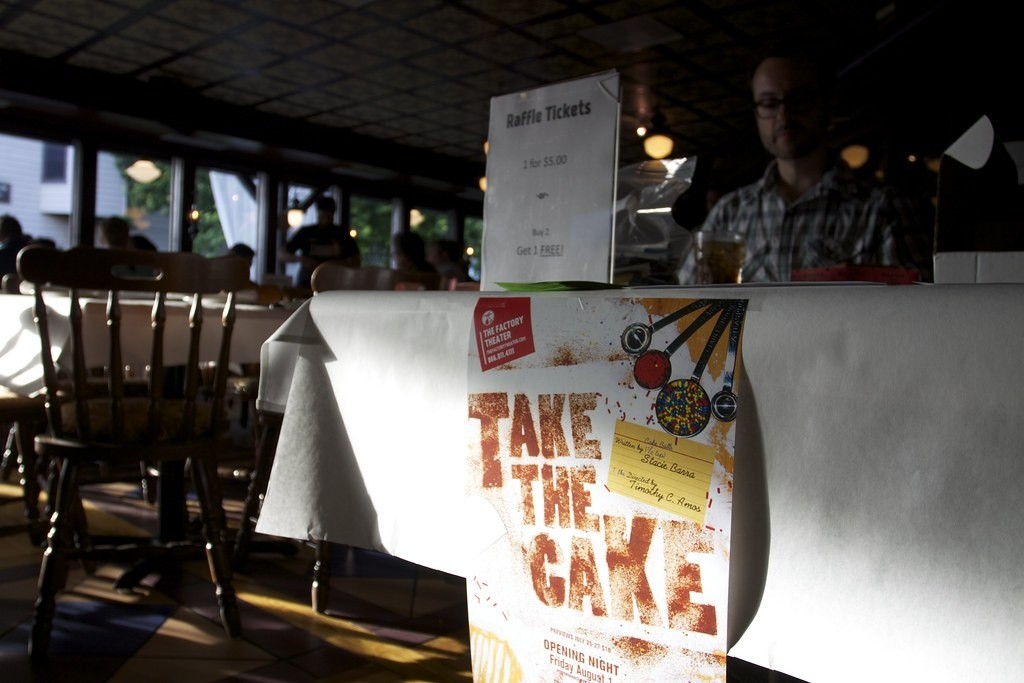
[391,233,437,291]
[674,50,935,283]
[427,240,464,290]
[0,215,158,290]
[279,198,360,287]
[688,186,719,233]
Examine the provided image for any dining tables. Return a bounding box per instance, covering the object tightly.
[247,281,1024,680]
[0,291,298,542]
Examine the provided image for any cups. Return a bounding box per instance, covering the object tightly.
[691,230,746,286]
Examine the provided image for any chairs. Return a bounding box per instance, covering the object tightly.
[14,241,257,677]
[0,392,99,580]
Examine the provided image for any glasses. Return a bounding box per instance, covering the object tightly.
[752,83,821,119]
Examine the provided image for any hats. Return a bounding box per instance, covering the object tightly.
[318,198,336,211]
